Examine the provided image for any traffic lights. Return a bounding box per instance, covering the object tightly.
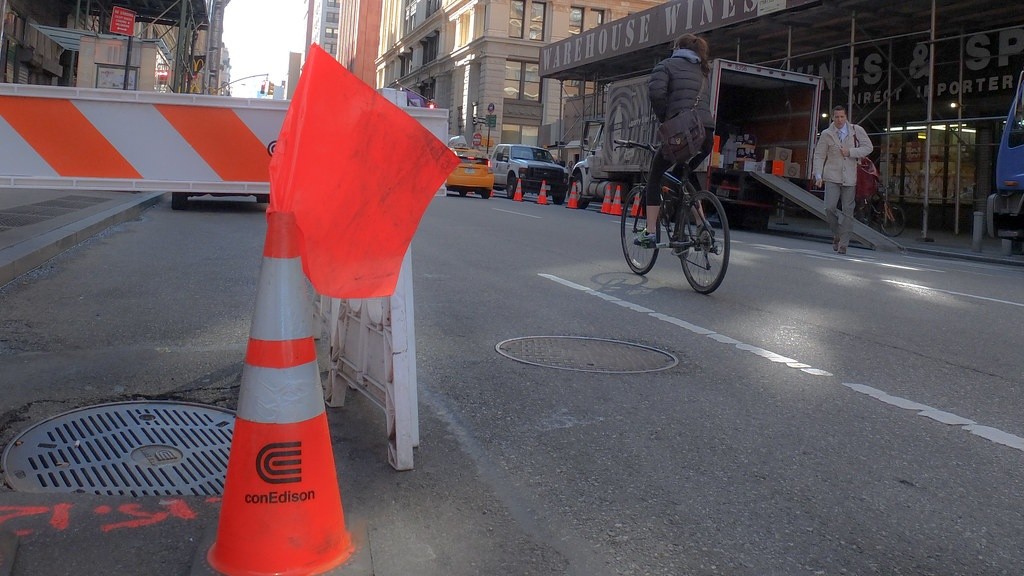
[428,102,437,109]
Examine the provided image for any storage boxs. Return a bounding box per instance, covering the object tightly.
[711,133,773,174]
[768,147,792,163]
[766,160,785,177]
[783,163,801,179]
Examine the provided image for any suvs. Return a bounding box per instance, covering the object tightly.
[491,143,569,205]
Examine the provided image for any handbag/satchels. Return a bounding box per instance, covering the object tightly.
[856,157,878,200]
[658,110,706,163]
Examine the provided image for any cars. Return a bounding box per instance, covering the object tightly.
[446,147,494,200]
[171,190,271,210]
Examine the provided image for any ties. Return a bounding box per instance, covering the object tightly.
[837,130,843,144]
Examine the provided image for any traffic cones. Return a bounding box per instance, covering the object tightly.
[512,179,523,201]
[565,181,578,209]
[608,185,621,215]
[601,185,611,214]
[205,209,353,576]
[537,179,546,204]
[629,192,644,219]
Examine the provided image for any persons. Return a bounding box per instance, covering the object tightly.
[632,32,716,249]
[565,160,574,172]
[811,104,874,254]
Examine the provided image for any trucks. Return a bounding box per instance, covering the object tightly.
[567,58,825,235]
[987,70,1024,237]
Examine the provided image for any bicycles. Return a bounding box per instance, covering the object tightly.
[611,138,732,295]
[856,175,906,238]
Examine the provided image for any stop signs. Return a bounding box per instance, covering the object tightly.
[472,132,482,146]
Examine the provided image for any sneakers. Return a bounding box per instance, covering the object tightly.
[696,220,711,237]
[633,229,656,249]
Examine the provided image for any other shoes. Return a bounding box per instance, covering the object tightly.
[838,246,846,254]
[833,239,839,251]
